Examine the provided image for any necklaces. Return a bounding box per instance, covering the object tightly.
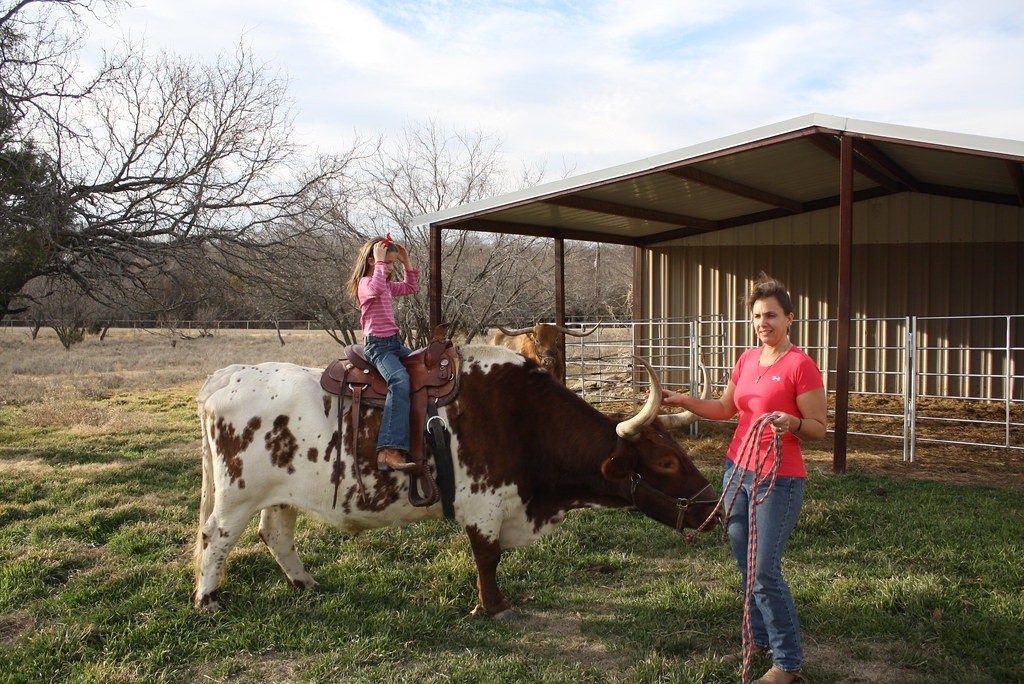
[756,341,791,385]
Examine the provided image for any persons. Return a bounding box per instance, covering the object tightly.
[646,281,826,684]
[346,236,420,471]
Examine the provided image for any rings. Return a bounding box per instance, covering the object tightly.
[779,427,782,432]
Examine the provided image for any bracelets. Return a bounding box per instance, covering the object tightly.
[374,261,387,265]
[791,418,802,435]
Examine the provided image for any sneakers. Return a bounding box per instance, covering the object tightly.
[720,642,774,667]
[749,666,801,684]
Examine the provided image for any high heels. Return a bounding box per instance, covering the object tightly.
[376,448,416,470]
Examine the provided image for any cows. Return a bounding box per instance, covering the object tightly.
[194,343,725,621]
[490,317,602,385]
